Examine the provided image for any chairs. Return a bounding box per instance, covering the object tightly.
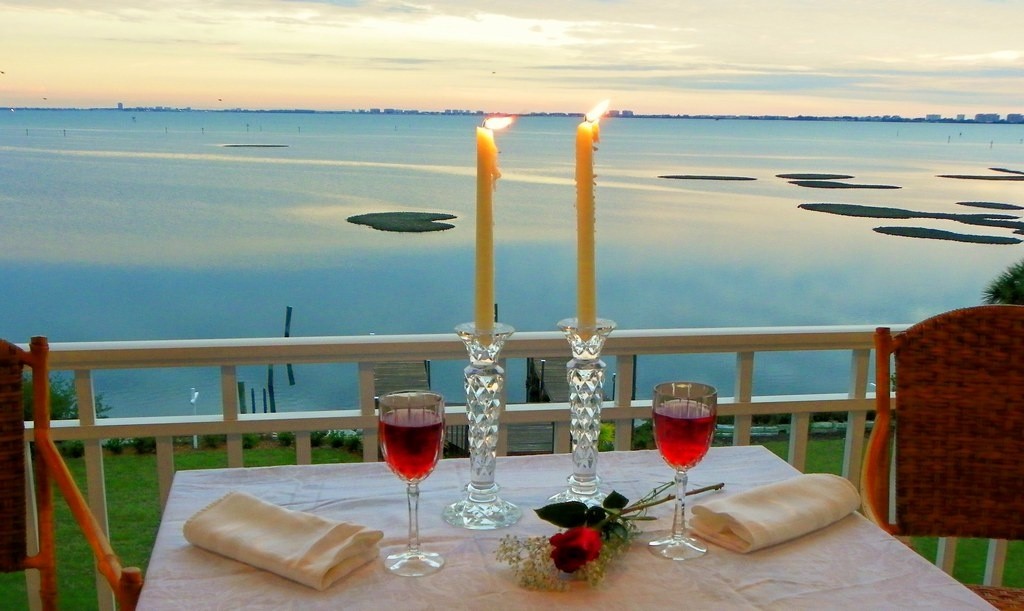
[0,336,143,611]
[862,304,1024,611]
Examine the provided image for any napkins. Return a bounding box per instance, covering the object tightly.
[183,489,384,593]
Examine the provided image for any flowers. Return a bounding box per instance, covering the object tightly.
[685,472,862,555]
[490,481,726,592]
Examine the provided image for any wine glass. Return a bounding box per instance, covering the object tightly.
[377,392,444,577]
[648,382,717,561]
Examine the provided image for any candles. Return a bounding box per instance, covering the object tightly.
[474,113,517,331]
[575,99,611,326]
